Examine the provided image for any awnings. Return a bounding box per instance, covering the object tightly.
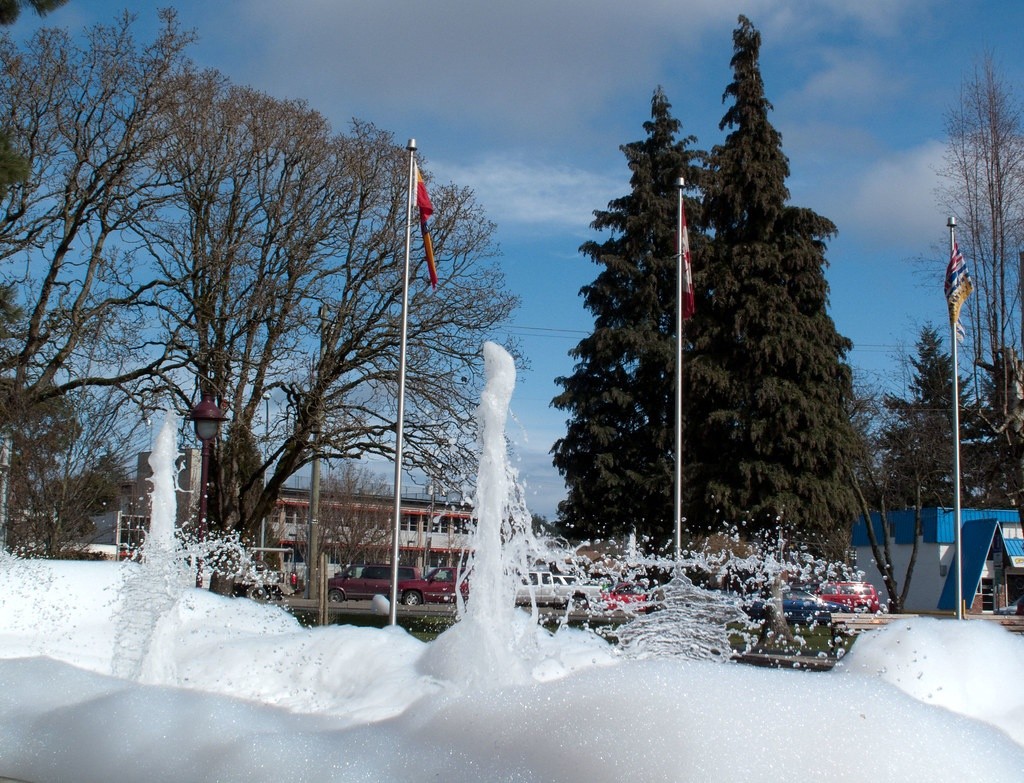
[1004,538,1024,569]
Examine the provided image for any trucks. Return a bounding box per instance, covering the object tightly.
[232,546,296,602]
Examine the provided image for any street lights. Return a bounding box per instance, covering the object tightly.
[180,388,231,587]
[259,393,270,560]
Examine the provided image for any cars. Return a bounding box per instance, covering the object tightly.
[993,596,1024,615]
[740,585,851,623]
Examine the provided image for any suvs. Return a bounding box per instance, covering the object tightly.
[803,580,880,615]
[327,564,422,604]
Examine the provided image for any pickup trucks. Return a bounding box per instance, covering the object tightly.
[516,570,604,612]
[583,582,658,612]
[388,567,472,606]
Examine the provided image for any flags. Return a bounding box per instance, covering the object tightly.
[416,164,438,289]
[681,198,695,319]
[944,242,974,343]
